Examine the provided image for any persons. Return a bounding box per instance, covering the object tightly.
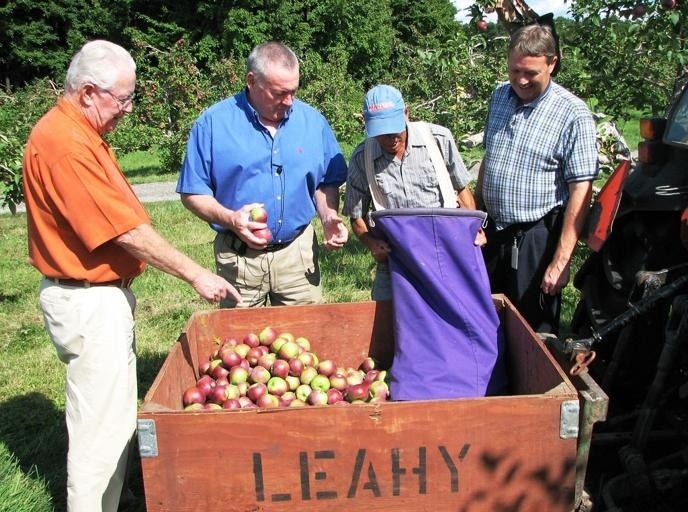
[23,39,245,512]
[340,85,488,302]
[176,39,350,310]
[473,23,601,358]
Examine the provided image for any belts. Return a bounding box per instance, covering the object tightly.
[46,276,134,288]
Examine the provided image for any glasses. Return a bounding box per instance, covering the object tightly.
[105,89,135,106]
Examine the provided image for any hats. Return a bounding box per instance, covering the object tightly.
[363,85,405,137]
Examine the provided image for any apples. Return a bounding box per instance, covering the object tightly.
[635,5,645,17]
[478,21,487,30]
[663,0,676,10]
[249,207,268,223]
[180,325,393,412]
[252,228,274,243]
